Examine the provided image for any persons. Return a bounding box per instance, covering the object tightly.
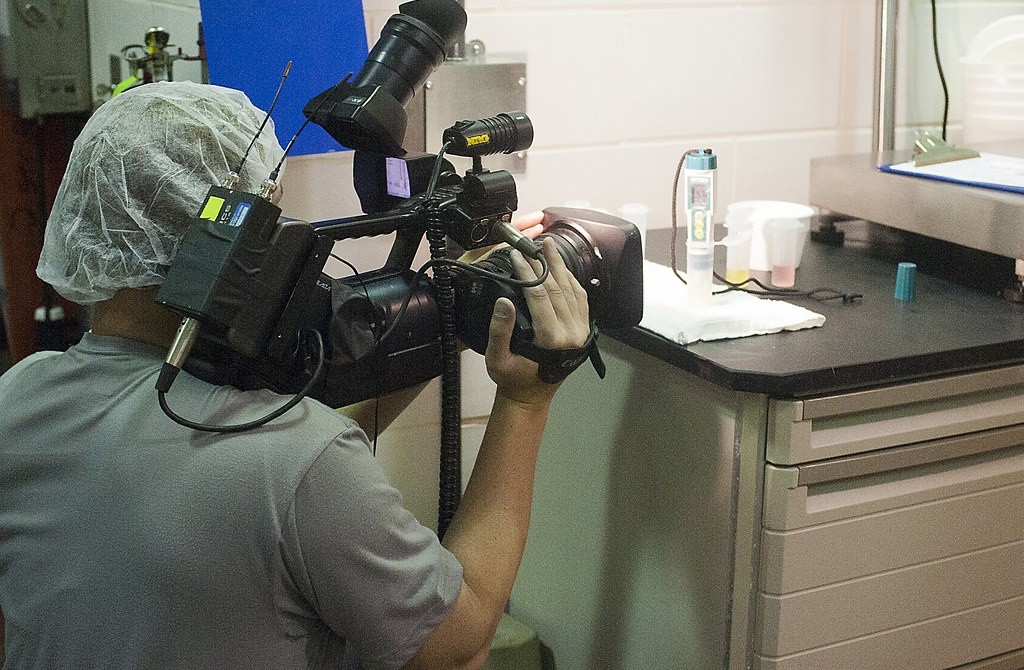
[0,82,587,667]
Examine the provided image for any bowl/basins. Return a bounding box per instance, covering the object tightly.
[727,200,815,271]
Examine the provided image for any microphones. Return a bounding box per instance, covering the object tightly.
[442,111,533,157]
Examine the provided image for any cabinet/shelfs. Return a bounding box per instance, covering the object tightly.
[502,217,1024,670]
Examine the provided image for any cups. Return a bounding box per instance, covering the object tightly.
[724,233,752,283]
[893,262,918,301]
[767,219,797,288]
